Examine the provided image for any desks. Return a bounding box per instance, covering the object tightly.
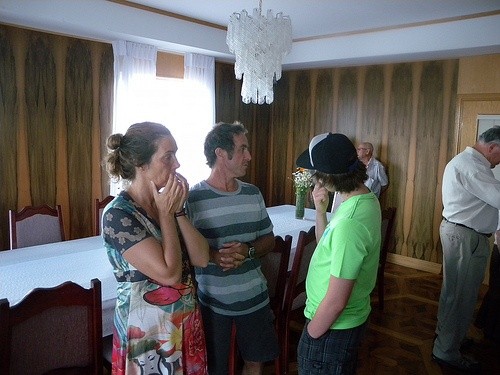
[0,203,332,338]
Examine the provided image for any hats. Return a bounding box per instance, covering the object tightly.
[296,132,359,173]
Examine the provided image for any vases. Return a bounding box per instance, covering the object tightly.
[294,187,308,220]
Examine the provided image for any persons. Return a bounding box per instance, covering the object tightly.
[295,131,382,375]
[356,142,389,200]
[186,121,276,375]
[100,122,210,375]
[430,127,500,366]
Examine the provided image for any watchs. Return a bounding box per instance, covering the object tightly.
[175,208,187,217]
[245,242,255,259]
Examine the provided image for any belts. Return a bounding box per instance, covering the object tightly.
[442,217,491,237]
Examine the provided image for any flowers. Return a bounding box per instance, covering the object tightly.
[289,168,313,217]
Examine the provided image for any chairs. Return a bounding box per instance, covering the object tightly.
[208,235,293,375]
[7,204,66,251]
[370,206,397,311]
[275,225,318,375]
[0,279,105,375]
[96,196,116,236]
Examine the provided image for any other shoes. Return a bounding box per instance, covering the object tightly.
[431,355,476,369]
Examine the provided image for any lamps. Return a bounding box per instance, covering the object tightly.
[225,0,293,106]
